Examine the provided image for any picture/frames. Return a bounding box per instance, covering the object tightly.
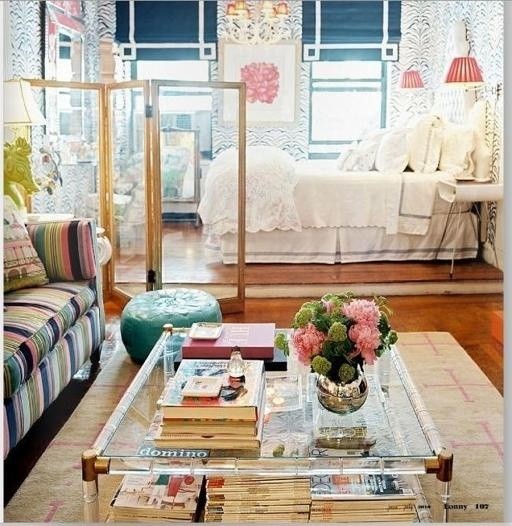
[219,38,302,129]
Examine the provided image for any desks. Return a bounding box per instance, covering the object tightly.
[81,324,456,525]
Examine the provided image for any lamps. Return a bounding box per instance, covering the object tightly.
[399,70,425,88]
[444,57,502,99]
[3,80,48,209]
[225,0,289,47]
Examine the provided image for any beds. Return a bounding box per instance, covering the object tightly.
[196,21,487,264]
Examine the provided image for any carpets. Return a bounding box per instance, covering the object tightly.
[3,331,505,525]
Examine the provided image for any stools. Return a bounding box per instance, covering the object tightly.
[120,287,223,365]
[86,192,132,227]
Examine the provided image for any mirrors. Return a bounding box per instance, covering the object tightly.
[160,125,201,228]
[56,23,84,140]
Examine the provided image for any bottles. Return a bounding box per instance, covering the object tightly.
[228,346,246,390]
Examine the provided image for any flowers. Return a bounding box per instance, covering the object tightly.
[274,291,398,386]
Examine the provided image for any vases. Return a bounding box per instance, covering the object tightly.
[317,374,368,414]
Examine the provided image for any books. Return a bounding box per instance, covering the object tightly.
[110,371,416,524]
[157,322,288,449]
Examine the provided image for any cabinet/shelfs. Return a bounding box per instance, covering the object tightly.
[435,180,504,278]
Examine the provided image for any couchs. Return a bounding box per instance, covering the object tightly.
[4,218,106,461]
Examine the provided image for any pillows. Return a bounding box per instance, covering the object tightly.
[408,114,442,173]
[375,129,410,174]
[3,195,49,292]
